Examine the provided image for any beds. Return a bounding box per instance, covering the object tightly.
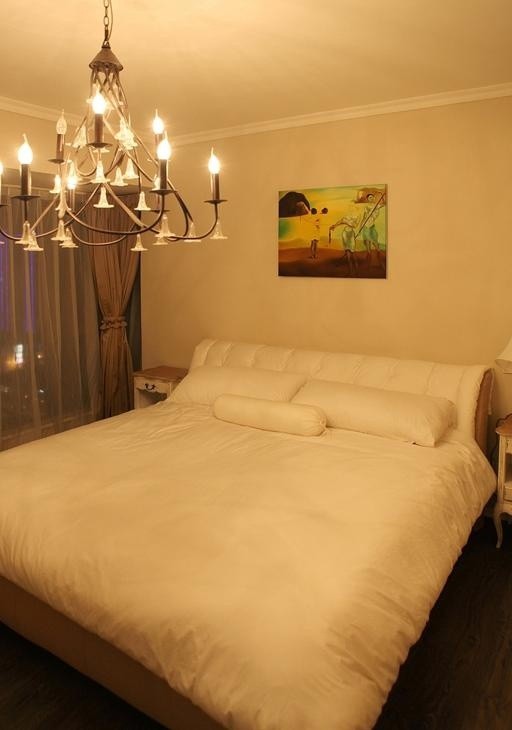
[0,335,493,730]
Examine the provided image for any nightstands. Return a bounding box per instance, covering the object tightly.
[134,365,188,408]
[493,415,512,548]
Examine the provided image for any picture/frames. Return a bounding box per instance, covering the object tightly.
[277,183,388,280]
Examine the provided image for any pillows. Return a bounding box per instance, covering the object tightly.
[215,392,326,437]
[169,365,306,406]
[292,379,456,448]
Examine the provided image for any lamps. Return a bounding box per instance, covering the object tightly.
[0,33,230,251]
